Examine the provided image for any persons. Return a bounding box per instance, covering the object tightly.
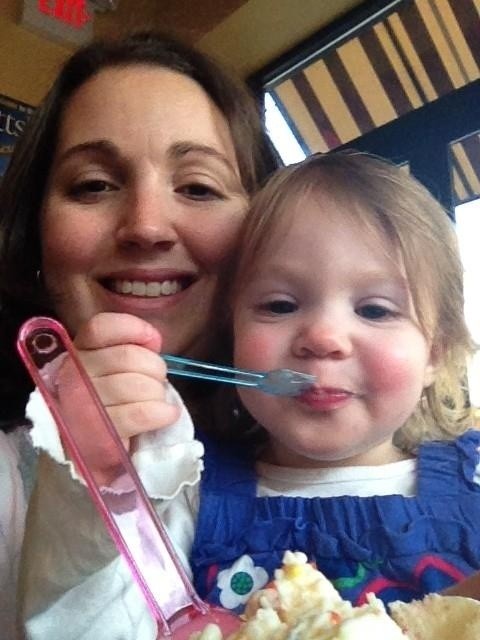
[23,148,480,639]
[0,28,271,639]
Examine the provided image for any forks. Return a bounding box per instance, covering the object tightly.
[159,352,319,398]
